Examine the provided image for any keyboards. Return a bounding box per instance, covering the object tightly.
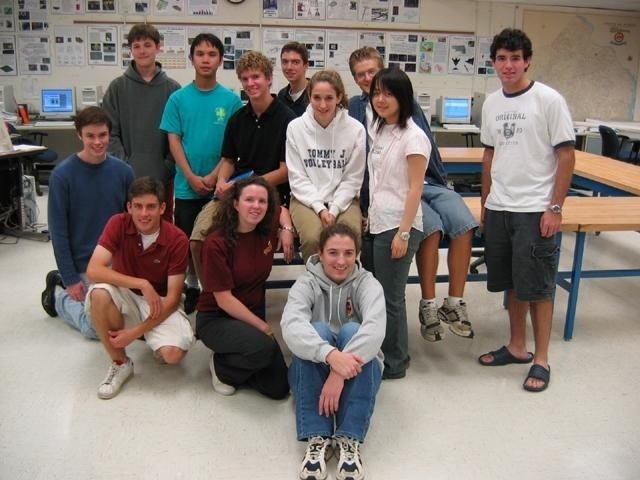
[443,124,479,130]
[35,122,74,127]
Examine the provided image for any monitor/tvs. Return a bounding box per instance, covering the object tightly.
[436,95,471,124]
[40,87,76,120]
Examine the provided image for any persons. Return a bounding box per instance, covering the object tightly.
[344,47,479,342]
[478,29,576,391]
[366,68,432,379]
[102,24,181,224]
[160,33,242,314]
[280,224,386,479]
[85,176,196,398]
[285,71,366,265]
[42,107,137,341]
[189,51,295,291]
[277,42,313,115]
[195,176,297,400]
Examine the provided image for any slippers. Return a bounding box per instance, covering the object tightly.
[479,346,534,366]
[523,360,550,392]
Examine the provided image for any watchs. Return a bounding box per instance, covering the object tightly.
[549,204,562,215]
[396,231,414,244]
[281,226,295,233]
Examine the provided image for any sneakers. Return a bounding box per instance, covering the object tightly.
[299,435,334,480]
[208,351,235,396]
[418,299,446,342]
[332,436,366,480]
[382,355,410,381]
[98,358,134,399]
[438,298,473,338]
[41,269,64,318]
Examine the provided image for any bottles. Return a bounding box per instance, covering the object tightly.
[18,105,28,123]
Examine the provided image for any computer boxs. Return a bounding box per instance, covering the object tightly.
[82,86,104,109]
[0,84,15,113]
[416,92,432,127]
[13,175,37,227]
